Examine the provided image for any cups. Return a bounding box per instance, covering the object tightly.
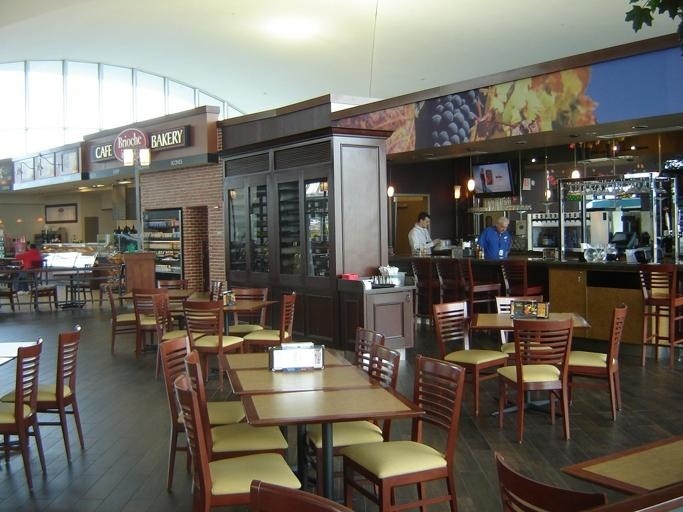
[473,197,480,209]
[484,197,513,211]
[504,205,532,210]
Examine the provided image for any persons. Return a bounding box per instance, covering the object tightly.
[11,235,61,298]
[475,216,513,260]
[408,211,440,255]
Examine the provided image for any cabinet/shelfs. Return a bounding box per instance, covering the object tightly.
[141,208,182,289]
[526,211,608,256]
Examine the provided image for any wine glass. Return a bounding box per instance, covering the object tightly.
[655,180,669,194]
[639,177,650,193]
[566,178,639,195]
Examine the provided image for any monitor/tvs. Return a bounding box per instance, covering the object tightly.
[471,161,517,197]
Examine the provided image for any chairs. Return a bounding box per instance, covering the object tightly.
[496,318,574,444]
[431,301,508,417]
[413,255,682,368]
[559,304,628,422]
[495,453,608,512]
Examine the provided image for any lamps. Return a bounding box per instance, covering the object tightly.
[387,158,394,198]
[572,143,580,178]
[453,159,461,202]
[544,169,552,201]
[467,155,475,192]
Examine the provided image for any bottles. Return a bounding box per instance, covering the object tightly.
[584,242,619,262]
[475,244,484,259]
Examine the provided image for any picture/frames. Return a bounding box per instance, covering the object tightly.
[45,203,77,223]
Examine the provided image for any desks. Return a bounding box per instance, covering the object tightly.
[561,435,683,494]
